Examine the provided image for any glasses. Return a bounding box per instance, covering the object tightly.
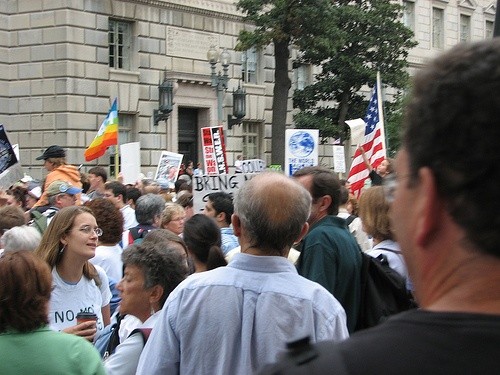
[48,181,72,197]
[170,216,185,223]
[382,173,417,202]
[64,226,103,237]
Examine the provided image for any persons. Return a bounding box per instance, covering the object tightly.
[291,167,375,336]
[0,224,43,252]
[33,205,112,344]
[103,239,192,375]
[0,145,301,266]
[339,179,358,216]
[359,144,395,185]
[0,249,106,375]
[183,213,228,274]
[82,198,124,317]
[257,34,500,375]
[358,186,414,293]
[337,186,371,253]
[133,172,350,375]
[110,228,189,343]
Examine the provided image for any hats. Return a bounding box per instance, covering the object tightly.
[27,186,42,199]
[19,176,33,182]
[35,145,67,160]
[46,179,85,200]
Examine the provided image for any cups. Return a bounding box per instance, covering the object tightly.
[76,312,97,342]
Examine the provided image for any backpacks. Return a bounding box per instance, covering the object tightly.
[358,250,417,331]
[93,313,127,361]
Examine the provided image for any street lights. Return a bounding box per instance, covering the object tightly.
[207,46,231,126]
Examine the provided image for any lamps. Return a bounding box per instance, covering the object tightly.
[153,67,174,125]
[227,75,248,130]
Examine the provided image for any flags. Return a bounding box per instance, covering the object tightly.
[83,97,117,162]
[345,82,385,201]
